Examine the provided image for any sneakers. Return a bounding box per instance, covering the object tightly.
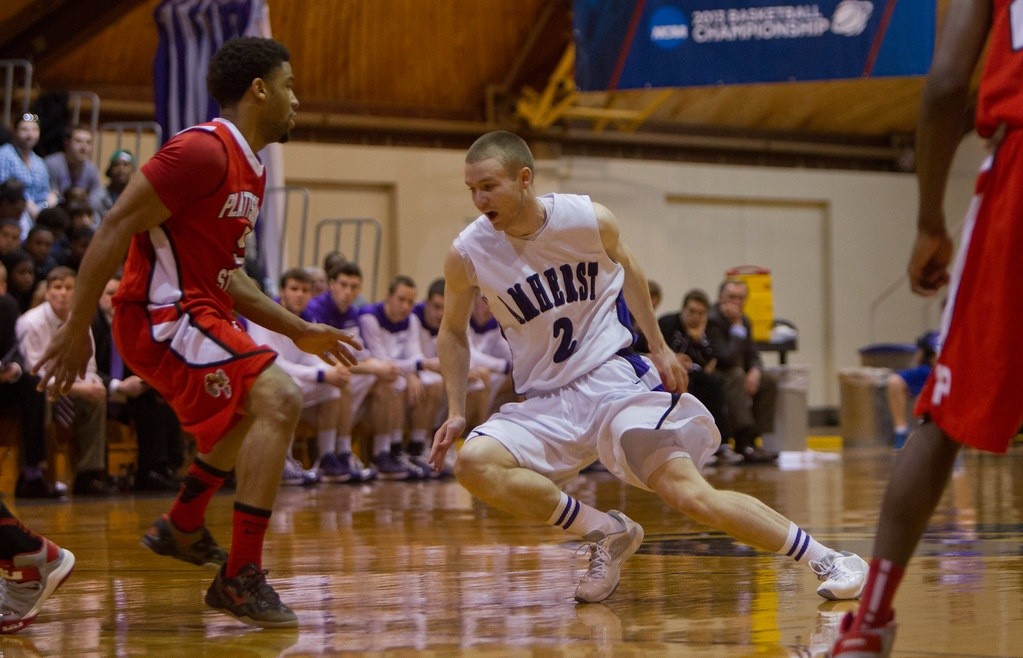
[0,537,75,633]
[576,605,623,658]
[206,562,298,628]
[142,516,228,564]
[829,611,898,658]
[810,601,860,652]
[809,550,870,599]
[574,510,644,603]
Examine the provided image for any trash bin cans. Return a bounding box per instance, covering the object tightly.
[757,365,808,451]
[839,367,893,447]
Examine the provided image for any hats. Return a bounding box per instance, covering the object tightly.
[106,149,136,177]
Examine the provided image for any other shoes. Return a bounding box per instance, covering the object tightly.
[136,462,179,491]
[741,445,777,462]
[14,473,66,499]
[278,446,456,485]
[892,427,917,451]
[73,472,131,495]
[717,443,744,464]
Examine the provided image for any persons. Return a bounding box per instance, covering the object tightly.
[833,0,1023,658]
[0,119,184,501]
[0,501,75,634]
[32,37,362,627]
[887,300,948,450]
[238,251,513,485]
[593,279,780,472]
[428,130,867,603]
